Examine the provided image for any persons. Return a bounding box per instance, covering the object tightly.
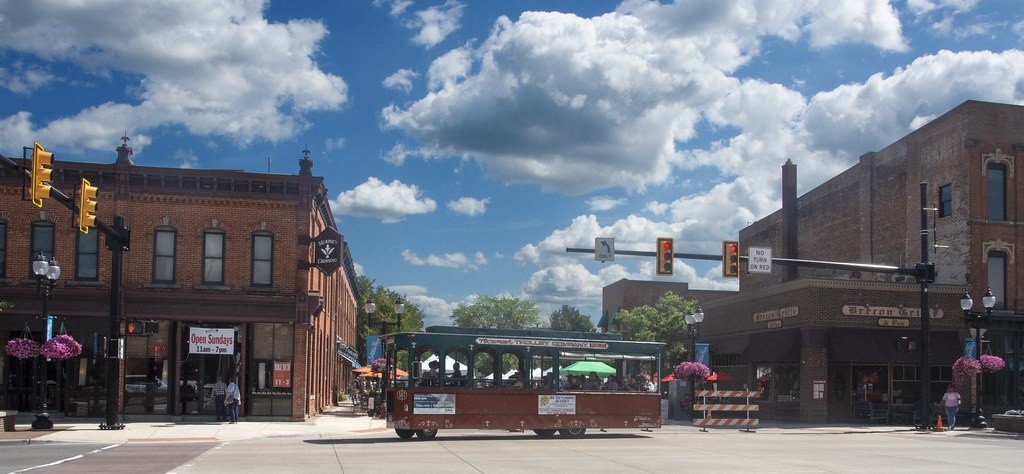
[211,376,230,422]
[417,360,461,387]
[225,378,240,424]
[502,371,657,393]
[939,384,961,430]
[680,394,693,419]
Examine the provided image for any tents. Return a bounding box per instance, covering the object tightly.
[482,361,617,383]
[421,353,467,376]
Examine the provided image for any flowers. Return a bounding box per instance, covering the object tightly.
[371,358,385,372]
[4,337,40,360]
[951,356,982,388]
[857,370,881,385]
[980,354,1005,370]
[39,334,83,360]
[673,360,711,384]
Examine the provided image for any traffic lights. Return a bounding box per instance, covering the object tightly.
[127,320,143,334]
[722,240,739,279]
[80,177,99,234]
[656,236,674,277]
[32,141,55,208]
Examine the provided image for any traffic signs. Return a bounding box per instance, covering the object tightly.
[594,237,614,262]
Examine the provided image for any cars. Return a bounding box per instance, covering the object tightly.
[179,380,199,402]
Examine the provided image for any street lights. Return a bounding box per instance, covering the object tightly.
[960,288,996,428]
[685,307,704,362]
[32,251,61,429]
[364,293,404,419]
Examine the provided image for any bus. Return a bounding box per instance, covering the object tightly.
[378,326,672,441]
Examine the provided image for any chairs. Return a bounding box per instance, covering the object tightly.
[349,395,367,416]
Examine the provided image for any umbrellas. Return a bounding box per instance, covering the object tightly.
[660,369,744,388]
[350,364,409,392]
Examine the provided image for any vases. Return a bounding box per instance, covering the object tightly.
[856,382,883,402]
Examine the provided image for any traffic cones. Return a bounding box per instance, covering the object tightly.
[934,416,943,432]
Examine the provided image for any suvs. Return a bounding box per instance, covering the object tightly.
[123,374,168,396]
